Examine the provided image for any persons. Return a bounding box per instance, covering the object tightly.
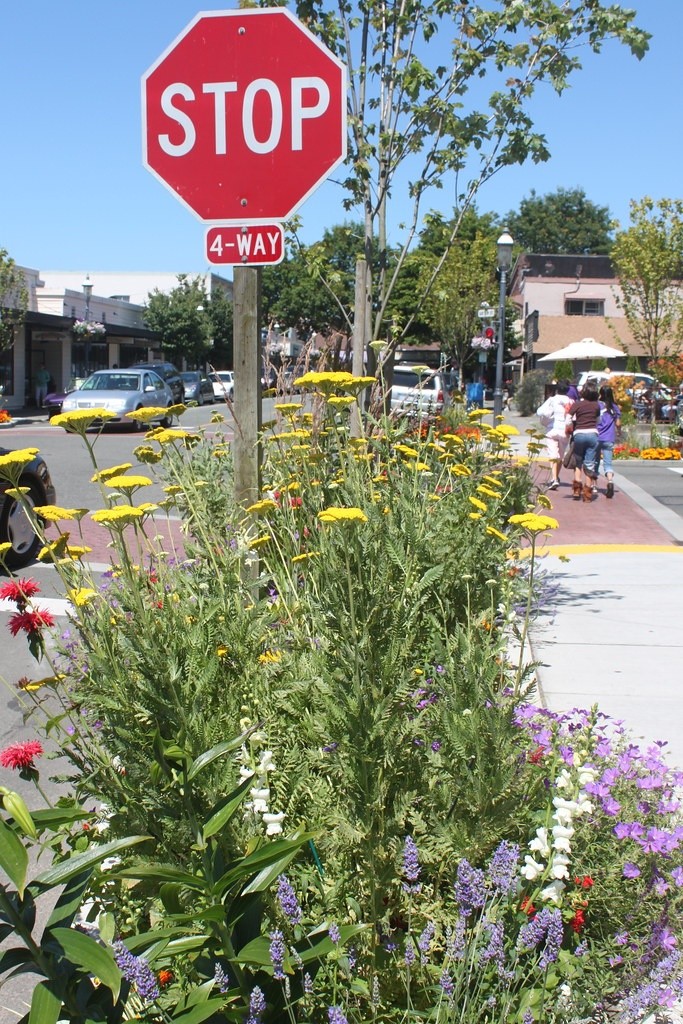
[630,380,683,424]
[538,381,621,501]
[465,375,519,413]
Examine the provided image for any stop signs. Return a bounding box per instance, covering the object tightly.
[139,7,351,226]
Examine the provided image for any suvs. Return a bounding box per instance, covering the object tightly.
[118,359,186,418]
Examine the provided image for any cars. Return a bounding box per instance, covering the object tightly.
[200,371,234,402]
[179,371,215,405]
[61,368,174,432]
[261,365,318,395]
[41,377,90,409]
[0,447,57,570]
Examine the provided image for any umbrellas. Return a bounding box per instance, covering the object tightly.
[538,338,628,370]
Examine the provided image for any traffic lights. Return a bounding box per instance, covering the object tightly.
[484,327,494,339]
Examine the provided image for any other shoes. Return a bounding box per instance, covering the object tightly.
[591,485,598,494]
[548,479,560,490]
[606,481,614,498]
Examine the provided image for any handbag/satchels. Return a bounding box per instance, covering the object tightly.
[562,443,576,469]
[540,396,555,426]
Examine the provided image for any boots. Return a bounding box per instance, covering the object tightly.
[572,481,582,498]
[583,484,592,503]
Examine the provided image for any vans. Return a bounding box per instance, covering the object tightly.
[375,366,456,420]
[575,371,678,425]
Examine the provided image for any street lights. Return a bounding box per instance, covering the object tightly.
[78,274,93,379]
[491,222,515,431]
